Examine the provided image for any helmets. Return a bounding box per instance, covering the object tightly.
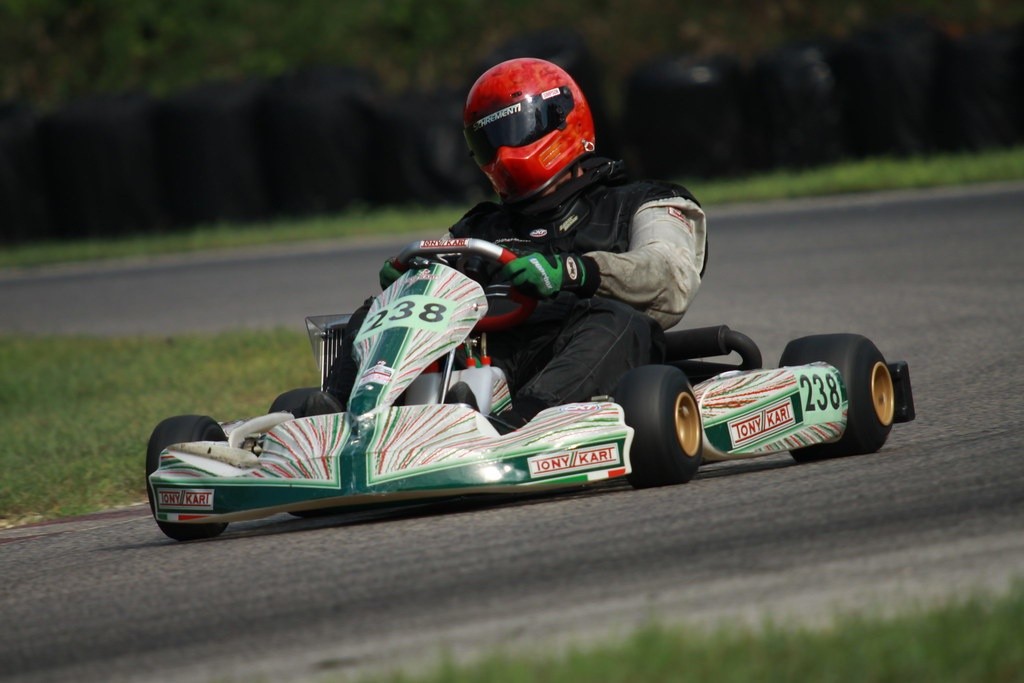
[463,57,595,206]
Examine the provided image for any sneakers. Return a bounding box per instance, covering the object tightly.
[305,390,346,418]
[443,381,479,416]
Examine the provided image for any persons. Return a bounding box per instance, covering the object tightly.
[329,56,709,451]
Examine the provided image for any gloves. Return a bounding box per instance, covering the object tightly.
[377,255,431,291]
[497,251,587,302]
